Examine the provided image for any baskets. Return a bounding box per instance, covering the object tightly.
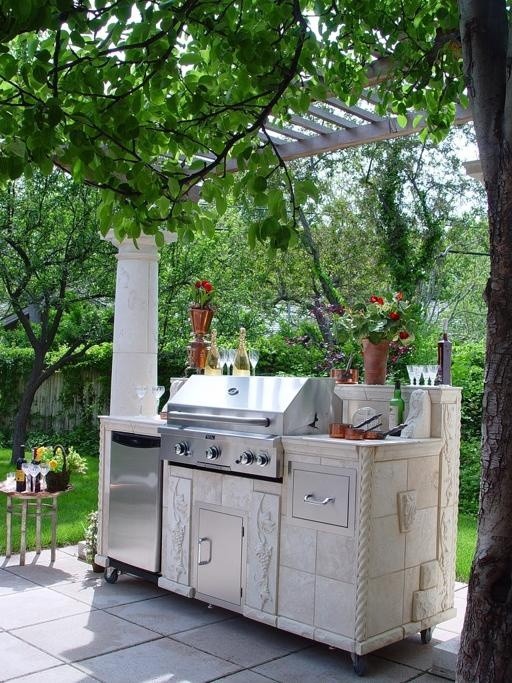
[45,444,70,492]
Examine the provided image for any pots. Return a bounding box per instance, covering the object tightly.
[329,413,383,438]
[331,352,360,385]
[345,423,382,440]
[365,423,408,440]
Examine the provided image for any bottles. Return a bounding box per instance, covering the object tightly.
[16,445,26,492]
[388,379,405,431]
[438,328,453,386]
[203,328,223,375]
[233,327,251,376]
[30,447,42,492]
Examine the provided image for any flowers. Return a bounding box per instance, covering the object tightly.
[36,446,88,475]
[193,279,218,309]
[339,292,426,345]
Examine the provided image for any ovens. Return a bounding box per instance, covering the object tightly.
[157,372,343,480]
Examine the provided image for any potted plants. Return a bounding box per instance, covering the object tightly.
[85,511,104,573]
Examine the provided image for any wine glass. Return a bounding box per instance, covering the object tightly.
[151,386,166,420]
[21,463,50,492]
[134,385,148,418]
[405,363,440,386]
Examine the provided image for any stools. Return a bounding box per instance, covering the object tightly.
[0,481,73,565]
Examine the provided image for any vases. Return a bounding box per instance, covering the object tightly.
[360,338,391,383]
[190,308,214,335]
